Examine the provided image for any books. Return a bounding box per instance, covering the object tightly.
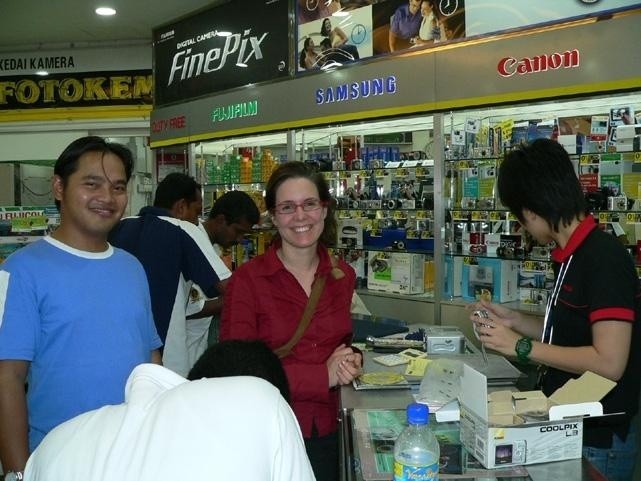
[351,358,450,390]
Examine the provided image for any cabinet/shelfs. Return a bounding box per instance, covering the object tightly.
[336,324,608,481]
[297,115,436,300]
[195,130,288,278]
[443,91,641,315]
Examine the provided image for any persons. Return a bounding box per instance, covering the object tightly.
[389,0,447,53]
[218,161,364,480]
[468,138,640,481]
[186,189,261,371]
[24,339,316,481]
[106,173,233,380]
[0,136,164,481]
[299,19,360,70]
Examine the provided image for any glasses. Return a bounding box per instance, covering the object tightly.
[273,199,330,215]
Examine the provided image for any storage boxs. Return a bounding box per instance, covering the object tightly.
[455,361,627,472]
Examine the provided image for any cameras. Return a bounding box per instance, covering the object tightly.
[327,158,434,272]
[495,443,512,465]
[473,310,492,338]
[451,106,641,305]
[512,440,526,464]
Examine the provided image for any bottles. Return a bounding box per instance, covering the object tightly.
[393,403,441,480]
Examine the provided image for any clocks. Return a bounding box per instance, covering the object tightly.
[424,140,435,161]
[350,23,368,44]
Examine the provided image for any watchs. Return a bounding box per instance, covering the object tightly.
[2,471,24,481]
[515,336,534,367]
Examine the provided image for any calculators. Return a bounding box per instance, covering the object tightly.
[373,348,427,367]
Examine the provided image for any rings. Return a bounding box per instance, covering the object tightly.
[344,355,349,361]
